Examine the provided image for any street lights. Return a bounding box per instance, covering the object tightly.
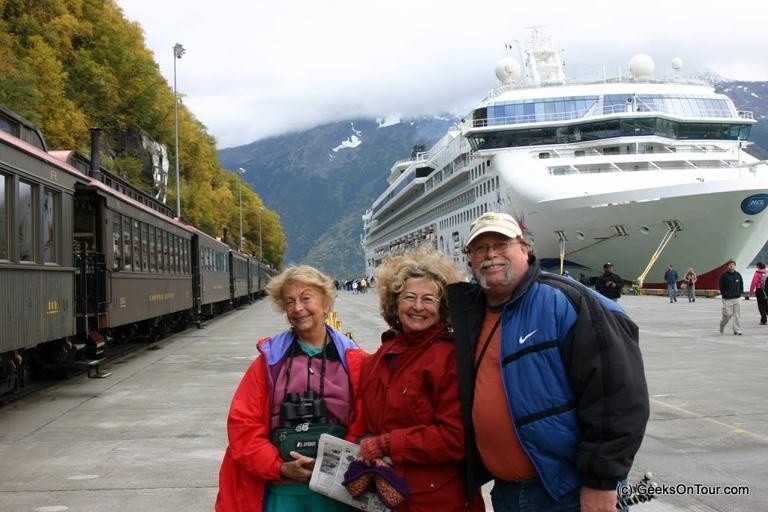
[173,43,184,218]
[259,206,264,258]
[237,167,246,251]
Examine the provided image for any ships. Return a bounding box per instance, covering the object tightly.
[358,25,768,298]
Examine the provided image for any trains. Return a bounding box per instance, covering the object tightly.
[0,130,280,393]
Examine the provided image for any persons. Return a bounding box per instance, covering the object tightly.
[595,262,623,303]
[381,212,650,512]
[761,272,768,300]
[719,259,743,335]
[750,262,768,325]
[356,242,487,511]
[333,277,372,294]
[580,274,591,288]
[562,271,574,281]
[215,262,371,511]
[664,264,678,303]
[683,268,697,302]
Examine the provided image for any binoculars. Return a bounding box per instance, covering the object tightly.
[280,390,328,427]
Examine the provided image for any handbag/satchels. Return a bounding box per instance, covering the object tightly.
[273,425,348,461]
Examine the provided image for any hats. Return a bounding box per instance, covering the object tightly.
[342,455,408,509]
[603,263,613,268]
[466,213,523,247]
